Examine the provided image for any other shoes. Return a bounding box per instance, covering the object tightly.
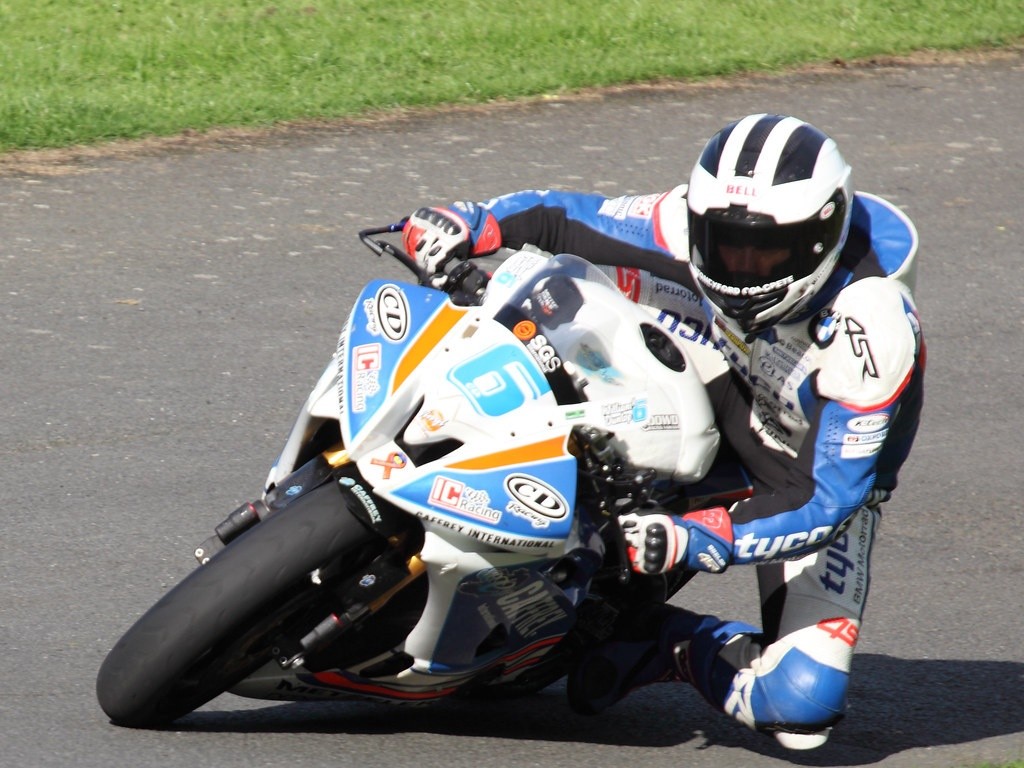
[565,603,698,713]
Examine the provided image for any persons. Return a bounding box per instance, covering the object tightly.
[402,113,927,749]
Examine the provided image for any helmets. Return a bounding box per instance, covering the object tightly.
[683,117,853,337]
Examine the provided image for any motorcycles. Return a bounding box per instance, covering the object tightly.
[94,188,920,730]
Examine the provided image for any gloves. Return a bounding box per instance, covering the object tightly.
[398,207,506,271]
[618,506,733,576]
